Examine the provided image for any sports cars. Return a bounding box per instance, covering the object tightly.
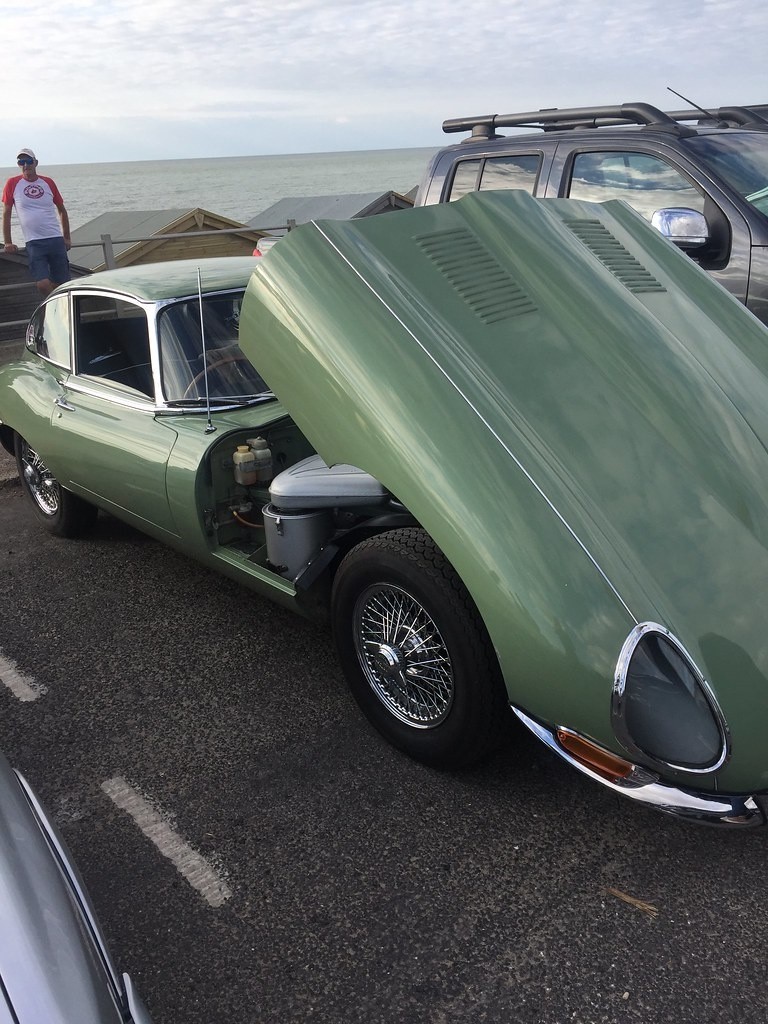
[0,188,767,841]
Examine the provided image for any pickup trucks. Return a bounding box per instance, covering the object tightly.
[253,98,768,343]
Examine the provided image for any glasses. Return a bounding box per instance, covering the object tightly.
[18,159,35,166]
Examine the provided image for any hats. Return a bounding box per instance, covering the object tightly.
[16,149,35,159]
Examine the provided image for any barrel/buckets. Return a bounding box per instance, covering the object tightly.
[262,501,334,580]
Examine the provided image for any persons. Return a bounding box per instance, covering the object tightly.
[2,148,72,297]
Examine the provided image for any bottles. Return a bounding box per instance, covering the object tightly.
[232,446,256,485]
[250,437,273,481]
